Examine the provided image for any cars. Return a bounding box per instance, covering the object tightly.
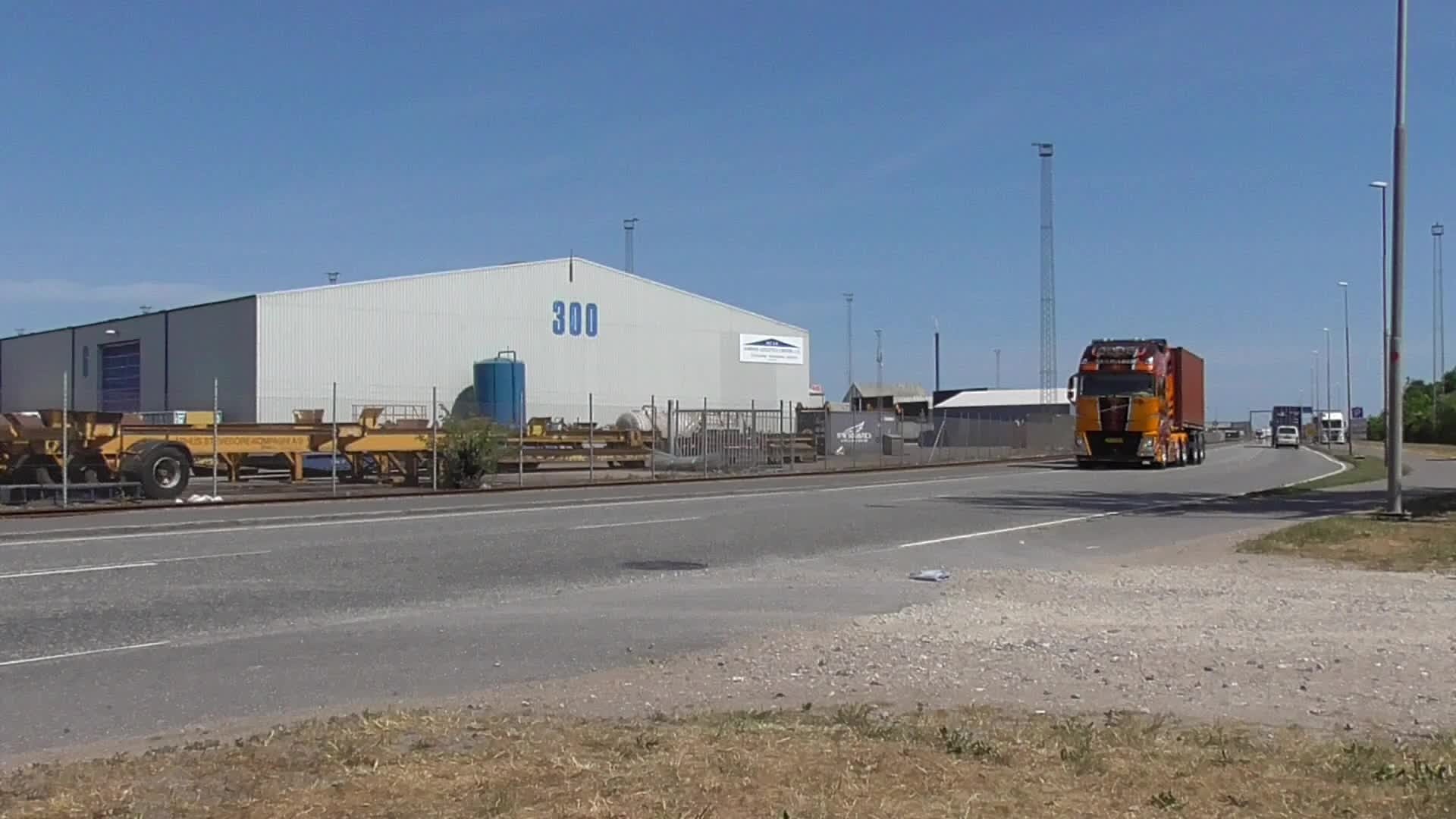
[293,450,355,479]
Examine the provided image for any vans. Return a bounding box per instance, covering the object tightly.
[1274,425,1300,450]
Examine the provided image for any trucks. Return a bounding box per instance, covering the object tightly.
[1269,406,1302,444]
[1069,339,1208,469]
[1316,411,1347,444]
[1255,429,1266,439]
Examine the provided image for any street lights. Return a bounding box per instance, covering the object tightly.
[1337,282,1353,457]
[843,291,854,411]
[1368,180,1391,469]
[1431,221,1446,433]
[623,217,639,275]
[1322,327,1332,451]
